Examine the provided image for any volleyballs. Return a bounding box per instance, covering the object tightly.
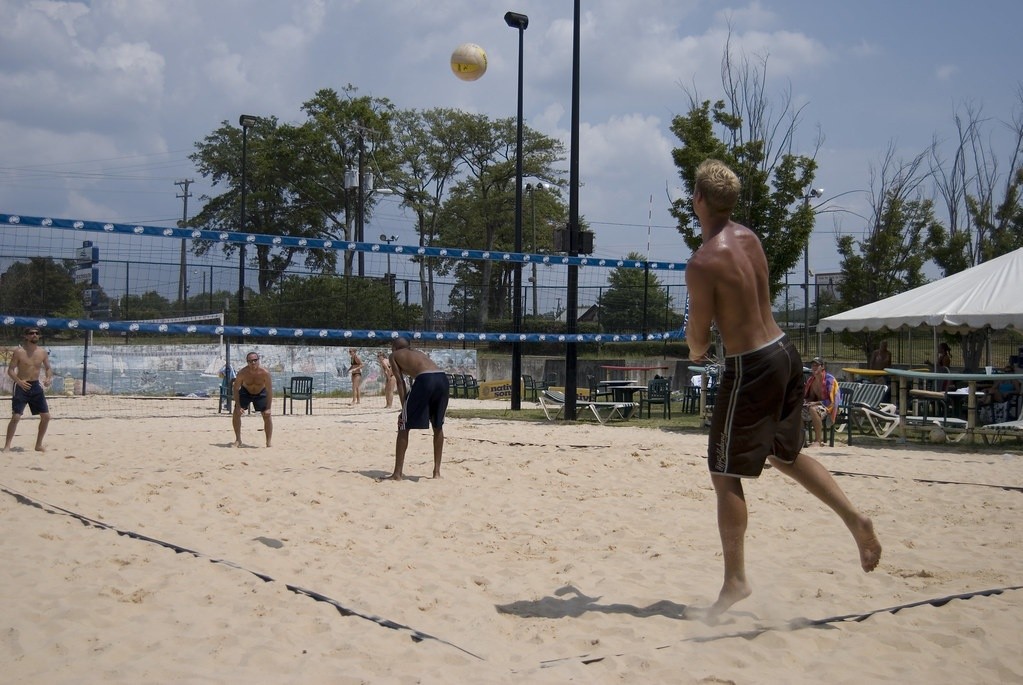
[929,429,946,445]
[450,43,488,81]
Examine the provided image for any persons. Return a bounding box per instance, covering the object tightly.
[984,366,1021,404]
[345,348,364,406]
[869,340,892,370]
[2,327,52,453]
[233,352,273,447]
[377,352,396,409]
[802,357,840,447]
[924,342,953,373]
[378,337,450,481]
[685,159,882,619]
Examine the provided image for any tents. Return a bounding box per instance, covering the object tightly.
[556,304,599,321]
[819,245,1023,418]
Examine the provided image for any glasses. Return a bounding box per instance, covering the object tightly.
[247,359,258,363]
[28,332,39,335]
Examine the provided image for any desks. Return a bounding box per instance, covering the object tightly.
[600,380,638,409]
[607,385,648,418]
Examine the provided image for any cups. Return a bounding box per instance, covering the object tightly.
[985,366,992,375]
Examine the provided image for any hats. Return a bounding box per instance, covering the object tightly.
[811,357,823,365]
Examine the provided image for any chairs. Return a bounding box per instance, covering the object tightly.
[218,378,251,415]
[534,390,640,426]
[905,416,968,445]
[464,375,481,399]
[808,388,854,446]
[641,379,672,421]
[454,374,467,399]
[587,375,614,410]
[446,374,456,399]
[834,382,900,438]
[282,376,313,415]
[681,375,713,416]
